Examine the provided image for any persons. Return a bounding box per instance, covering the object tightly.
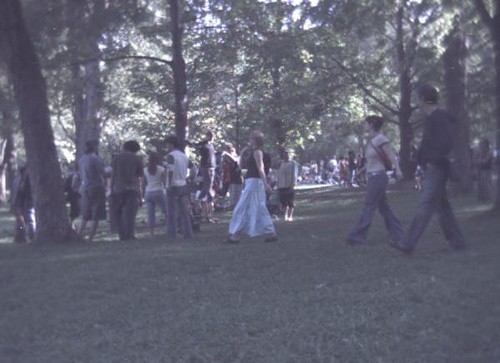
[344,115,408,246]
[9,127,500,244]
[389,84,471,254]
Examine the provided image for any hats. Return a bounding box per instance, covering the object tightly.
[283,149,290,153]
[85,136,101,146]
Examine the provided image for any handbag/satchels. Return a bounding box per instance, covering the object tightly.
[379,148,392,168]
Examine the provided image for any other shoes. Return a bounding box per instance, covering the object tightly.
[441,239,465,251]
[388,238,416,255]
[264,235,277,243]
[221,233,240,244]
